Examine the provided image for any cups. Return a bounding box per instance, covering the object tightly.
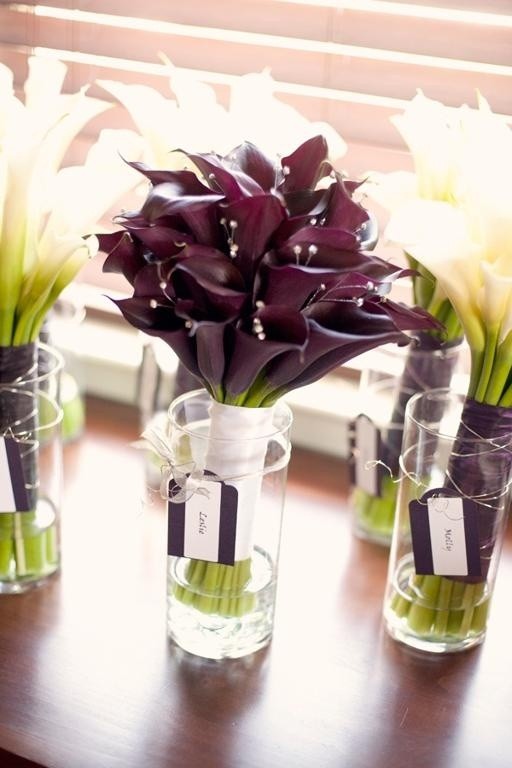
[380,385,511,659]
[1,289,87,595]
[138,330,211,498]
[345,333,467,553]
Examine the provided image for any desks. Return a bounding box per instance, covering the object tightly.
[0,393,511,768]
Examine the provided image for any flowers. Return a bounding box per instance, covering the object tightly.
[0,51,512,642]
